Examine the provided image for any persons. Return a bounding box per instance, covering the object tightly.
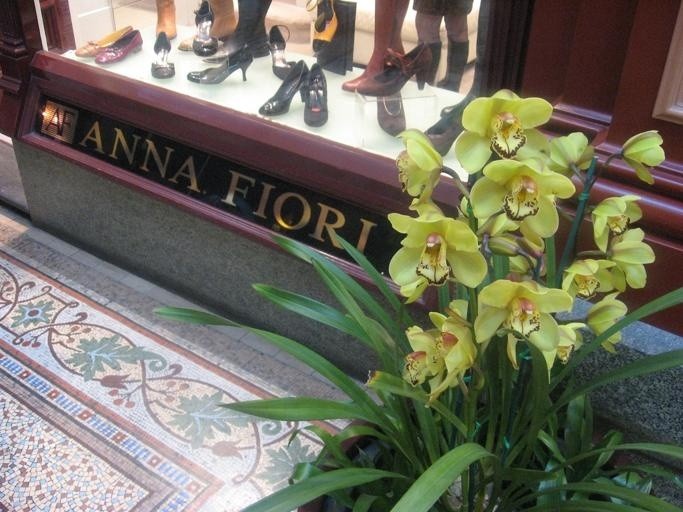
[413,1,474,91]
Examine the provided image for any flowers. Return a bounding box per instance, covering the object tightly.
[152,89,683,512]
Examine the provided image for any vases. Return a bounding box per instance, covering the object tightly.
[299,401,448,512]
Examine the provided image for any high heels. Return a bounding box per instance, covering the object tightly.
[187,44,252,84]
[259,59,328,128]
[358,42,433,95]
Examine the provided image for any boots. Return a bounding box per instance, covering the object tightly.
[178,0,272,64]
[428,40,469,92]
[342,0,410,92]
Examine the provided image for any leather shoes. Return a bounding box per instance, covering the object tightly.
[424,96,473,157]
[155,1,177,39]
[75,26,143,65]
[377,92,406,136]
[270,23,297,79]
[151,31,175,78]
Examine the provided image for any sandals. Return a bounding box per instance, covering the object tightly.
[305,0,338,52]
